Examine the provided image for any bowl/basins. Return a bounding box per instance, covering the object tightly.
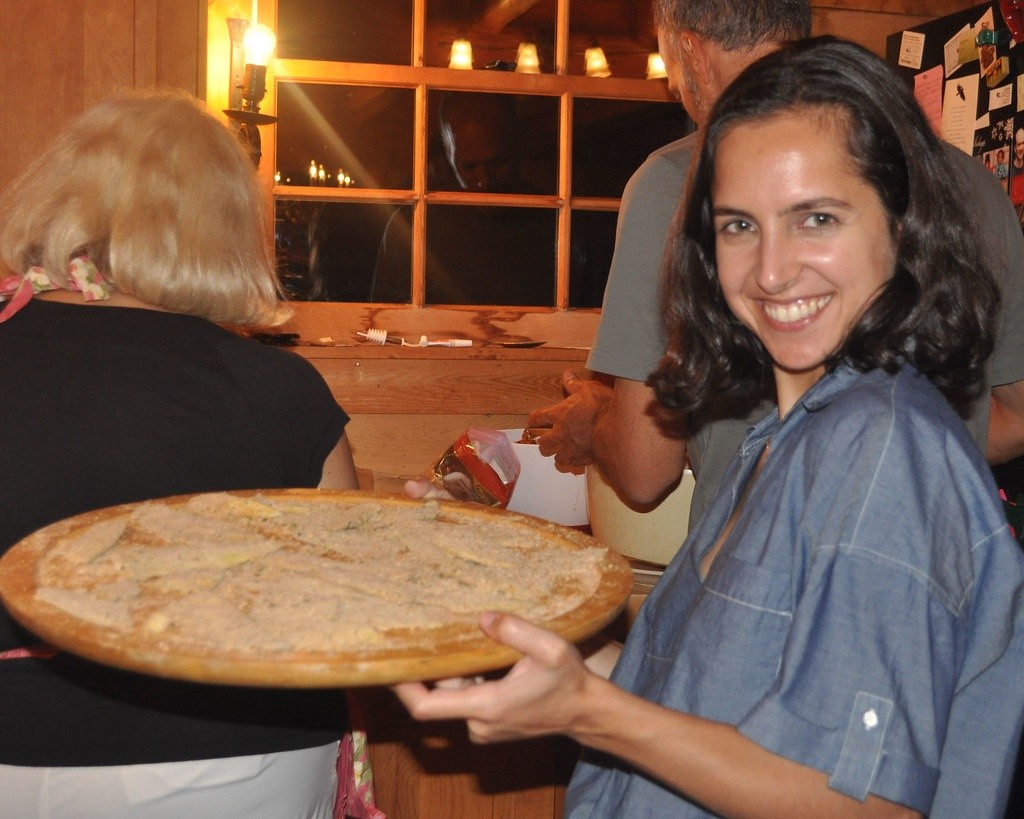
[585,459,696,568]
[493,428,591,527]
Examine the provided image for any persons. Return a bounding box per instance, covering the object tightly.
[995,150,1006,178]
[0,91,363,818]
[370,93,587,308]
[985,154,993,173]
[1013,128,1024,175]
[391,32,1024,819]
[527,0,1024,514]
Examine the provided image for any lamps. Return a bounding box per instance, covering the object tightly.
[644,44,669,80]
[449,30,475,70]
[226,19,283,171]
[517,41,541,75]
[272,157,359,299]
[585,38,612,78]
